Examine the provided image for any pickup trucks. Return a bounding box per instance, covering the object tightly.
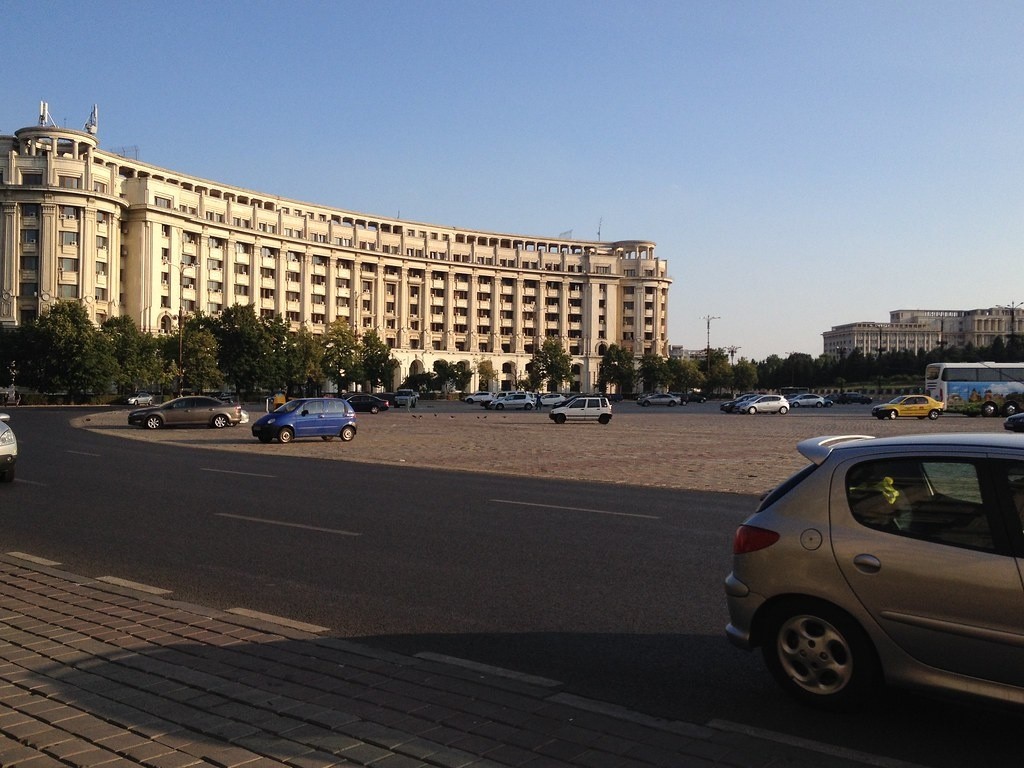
[393,389,416,408]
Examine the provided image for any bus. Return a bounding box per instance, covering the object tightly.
[925,362,1024,417]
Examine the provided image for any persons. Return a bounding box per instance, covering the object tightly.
[534,392,542,410]
[14,390,21,407]
[4,392,10,407]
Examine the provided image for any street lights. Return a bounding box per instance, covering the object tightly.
[699,314,721,373]
[162,260,200,398]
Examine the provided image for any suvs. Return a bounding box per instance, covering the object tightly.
[480,391,538,410]
[724,430,1024,716]
[464,392,496,404]
[372,392,393,404]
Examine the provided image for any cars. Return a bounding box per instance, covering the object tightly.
[824,392,872,405]
[671,392,706,406]
[0,412,17,483]
[121,392,153,406]
[540,393,565,407]
[338,392,389,414]
[252,398,357,444]
[720,393,760,414]
[593,393,624,402]
[637,393,681,407]
[128,395,242,430]
[872,395,945,420]
[552,393,603,409]
[414,392,420,400]
[734,394,790,415]
[785,393,798,400]
[1004,412,1024,433]
[202,391,249,427]
[787,393,825,408]
[549,395,612,424]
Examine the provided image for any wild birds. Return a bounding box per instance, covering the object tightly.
[411,413,507,419]
